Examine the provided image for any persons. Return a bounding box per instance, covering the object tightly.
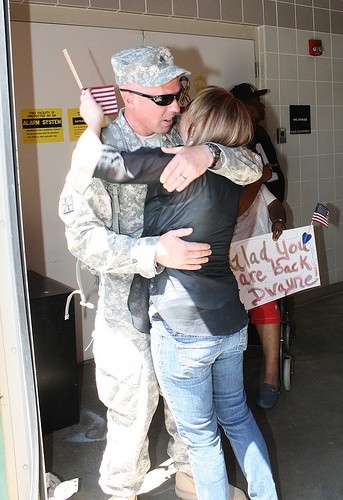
[59,46,262,500]
[72,84,277,500]
[230,82,287,409]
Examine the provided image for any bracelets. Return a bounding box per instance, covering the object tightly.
[273,218,285,224]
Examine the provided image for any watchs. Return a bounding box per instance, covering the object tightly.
[206,143,222,170]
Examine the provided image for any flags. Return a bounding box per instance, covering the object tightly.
[312,203,330,228]
[82,85,119,115]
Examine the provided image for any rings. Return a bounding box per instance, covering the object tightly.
[180,175,186,181]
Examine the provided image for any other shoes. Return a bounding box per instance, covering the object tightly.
[108,495,138,500]
[259,379,280,408]
[175,470,247,500]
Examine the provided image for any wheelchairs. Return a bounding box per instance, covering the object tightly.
[243,295,294,393]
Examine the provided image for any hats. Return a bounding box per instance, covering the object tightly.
[229,83,268,101]
[111,45,191,87]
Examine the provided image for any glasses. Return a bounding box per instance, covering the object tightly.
[119,88,183,106]
